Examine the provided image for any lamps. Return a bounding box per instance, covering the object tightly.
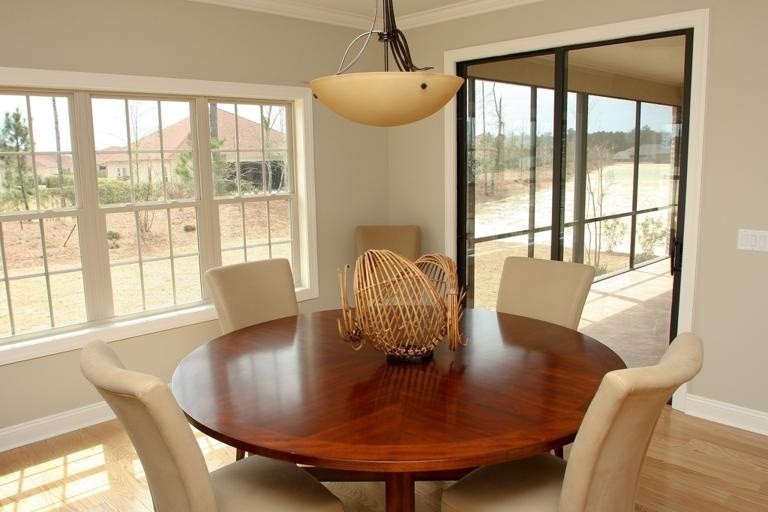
[307,1,467,127]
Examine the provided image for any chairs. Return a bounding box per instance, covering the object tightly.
[438,333,704,512]
[497,255,596,458]
[83,337,345,512]
[353,223,424,266]
[206,257,305,458]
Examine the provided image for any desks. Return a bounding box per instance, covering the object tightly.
[172,300,627,511]
